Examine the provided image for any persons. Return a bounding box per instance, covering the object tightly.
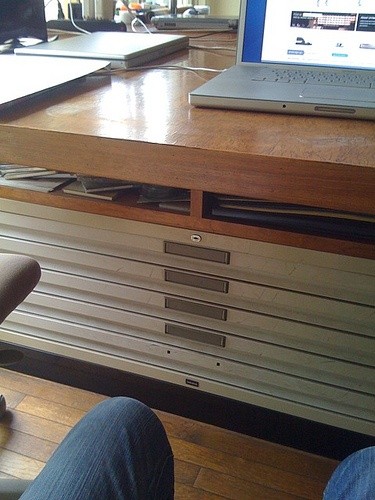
[19,396,375,500]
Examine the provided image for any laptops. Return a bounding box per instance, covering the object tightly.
[14,31,188,70]
[187,0,375,121]
[151,14,238,33]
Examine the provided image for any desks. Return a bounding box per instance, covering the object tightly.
[1,21,375,463]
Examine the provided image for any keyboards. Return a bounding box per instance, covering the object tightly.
[49,19,127,34]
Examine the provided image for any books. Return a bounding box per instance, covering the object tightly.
[0,163,375,246]
